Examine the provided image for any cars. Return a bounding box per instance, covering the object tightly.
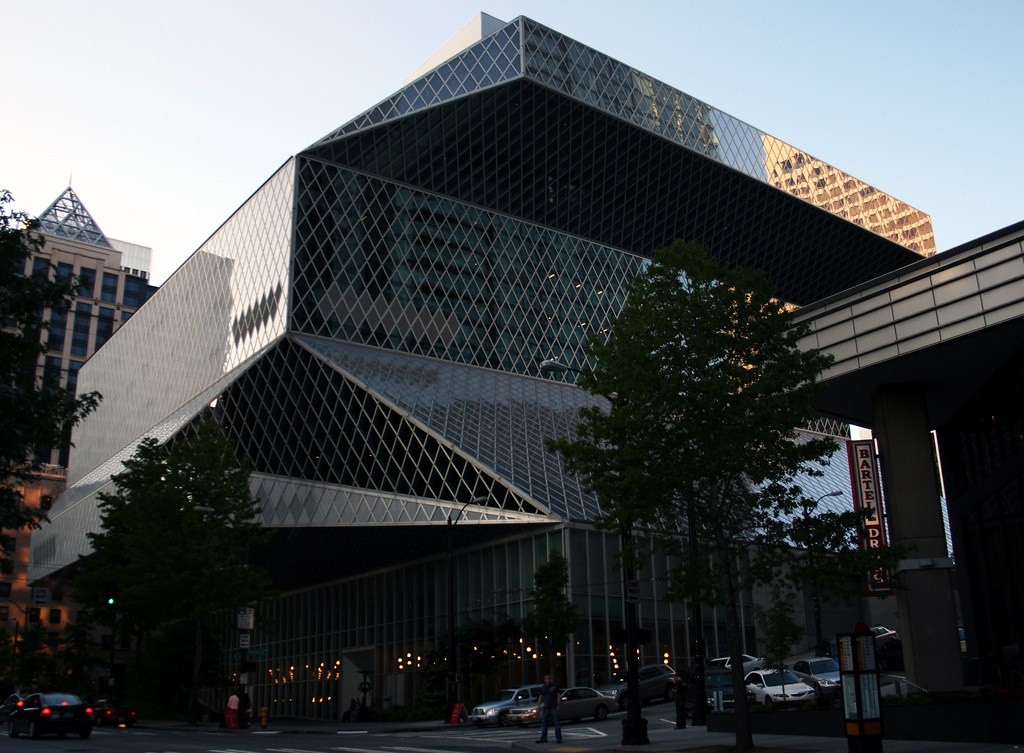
[674,664,757,716]
[870,625,896,647]
[783,654,844,705]
[7,693,94,739]
[709,653,769,676]
[91,698,138,730]
[505,686,620,727]
[743,668,816,711]
[0,692,32,725]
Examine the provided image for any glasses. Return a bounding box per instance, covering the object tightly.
[547,678,551,679]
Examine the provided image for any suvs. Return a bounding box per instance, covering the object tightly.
[468,684,546,728]
[597,663,679,710]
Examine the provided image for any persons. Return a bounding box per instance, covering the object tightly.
[672,674,688,729]
[341,699,356,723]
[227,690,251,729]
[535,673,562,744]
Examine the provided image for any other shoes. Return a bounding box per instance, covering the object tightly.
[557,739,562,743]
[536,739,547,743]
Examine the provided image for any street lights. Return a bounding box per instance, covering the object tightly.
[800,489,845,656]
[538,358,651,746]
[446,496,490,724]
